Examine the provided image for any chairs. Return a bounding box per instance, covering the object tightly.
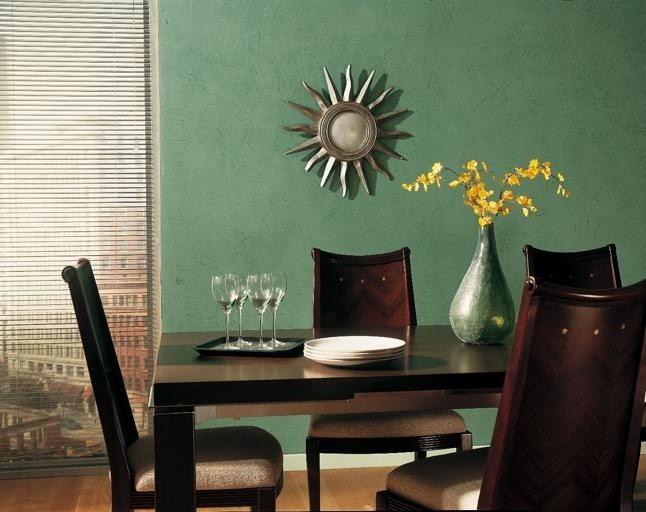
[376,276,646,512]
[305,247,472,511]
[61,258,284,512]
[522,243,622,290]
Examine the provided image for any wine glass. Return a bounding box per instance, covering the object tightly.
[209,272,286,353]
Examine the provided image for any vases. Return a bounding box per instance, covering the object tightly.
[449,222,516,345]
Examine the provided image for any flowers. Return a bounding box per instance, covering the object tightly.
[400,159,572,228]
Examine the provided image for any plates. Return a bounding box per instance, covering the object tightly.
[302,334,405,369]
